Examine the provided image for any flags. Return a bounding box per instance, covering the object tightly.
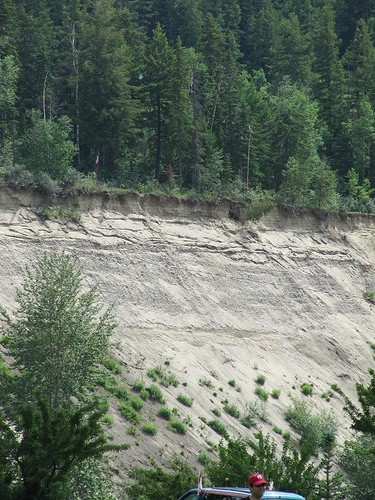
[95,149,101,168]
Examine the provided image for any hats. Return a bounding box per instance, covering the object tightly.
[248,474,267,486]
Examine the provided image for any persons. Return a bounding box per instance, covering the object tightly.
[239,472,269,500]
